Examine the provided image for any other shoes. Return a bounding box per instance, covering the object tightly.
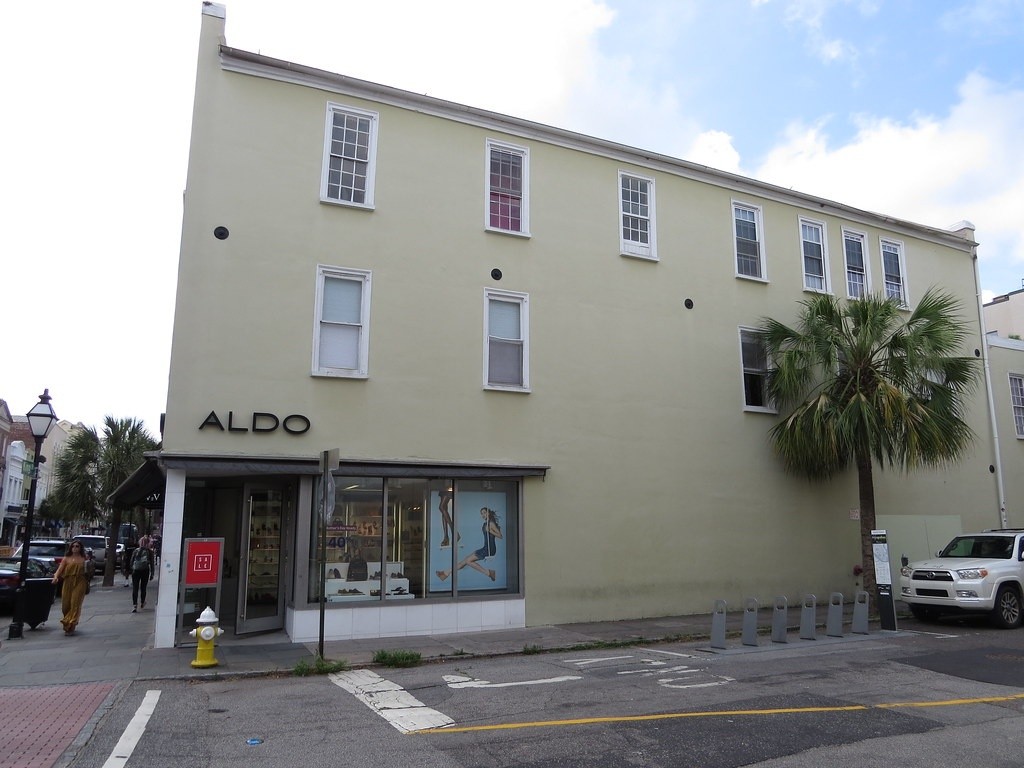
[436,570,447,581]
[457,534,461,543]
[441,540,449,547]
[65,631,73,636]
[328,568,408,596]
[489,569,496,582]
[141,602,146,609]
[132,606,136,613]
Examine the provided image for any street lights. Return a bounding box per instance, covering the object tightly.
[8,389,60,640]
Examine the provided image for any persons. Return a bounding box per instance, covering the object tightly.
[129,536,155,612]
[124,533,161,587]
[51,541,88,635]
[436,507,503,582]
[438,491,461,546]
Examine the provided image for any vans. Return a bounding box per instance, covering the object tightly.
[73,534,117,573]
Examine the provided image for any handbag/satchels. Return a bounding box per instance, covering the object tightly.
[347,556,367,580]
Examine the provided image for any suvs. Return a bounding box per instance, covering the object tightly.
[899,528,1024,630]
[11,541,96,579]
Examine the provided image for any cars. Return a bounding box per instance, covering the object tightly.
[116,544,125,565]
[0,557,56,604]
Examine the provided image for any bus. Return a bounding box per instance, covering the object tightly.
[105,523,138,547]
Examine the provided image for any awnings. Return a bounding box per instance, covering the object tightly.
[106,443,164,511]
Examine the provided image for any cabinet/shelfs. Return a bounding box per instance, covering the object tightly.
[246,491,426,605]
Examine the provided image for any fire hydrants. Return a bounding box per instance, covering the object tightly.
[189,606,224,668]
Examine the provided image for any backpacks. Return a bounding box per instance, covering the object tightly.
[133,547,149,571]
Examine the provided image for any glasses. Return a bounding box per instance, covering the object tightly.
[71,544,81,548]
